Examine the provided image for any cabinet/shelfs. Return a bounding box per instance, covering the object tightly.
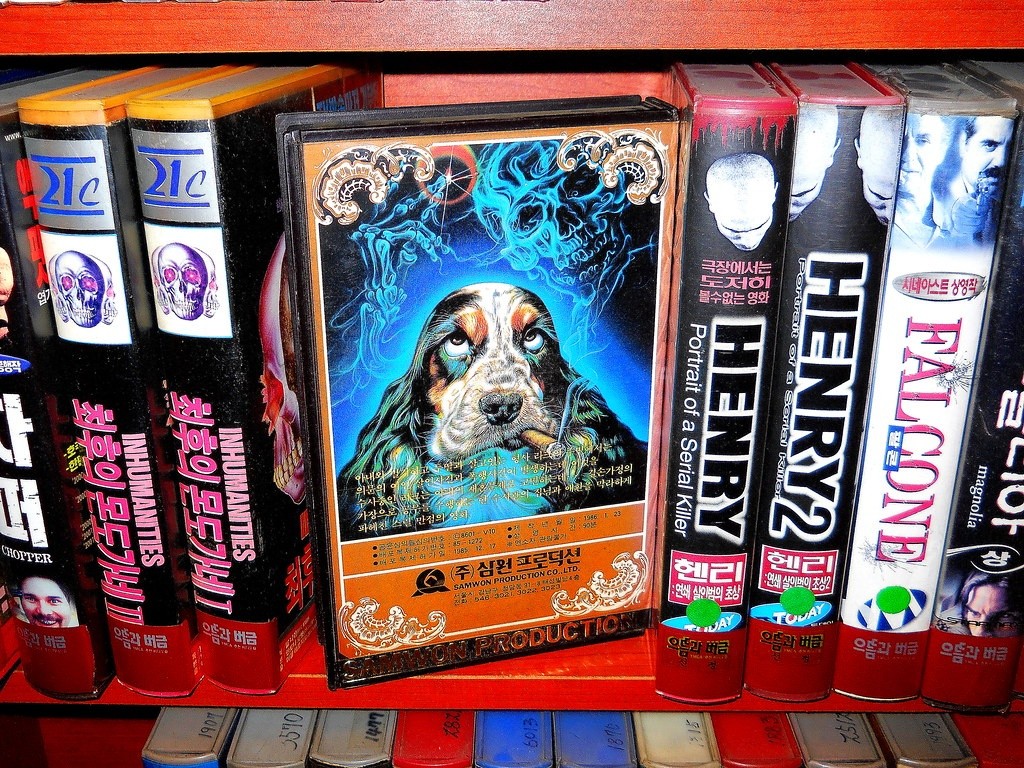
[0,0,1024,710]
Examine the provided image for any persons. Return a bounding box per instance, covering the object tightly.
[897,114,1015,252]
[704,152,780,252]
[787,101,842,222]
[16,571,73,628]
[961,572,1017,637]
[852,106,904,227]
